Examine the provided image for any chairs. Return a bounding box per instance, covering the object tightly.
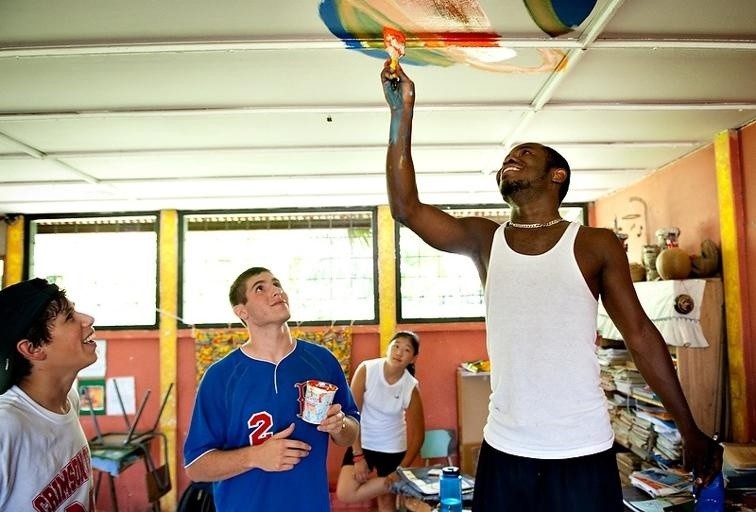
[83,379,174,502]
[420,429,457,466]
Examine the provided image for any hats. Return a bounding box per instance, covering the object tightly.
[1,278,58,397]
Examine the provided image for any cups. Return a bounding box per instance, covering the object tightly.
[301,379,339,426]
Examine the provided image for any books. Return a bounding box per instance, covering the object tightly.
[596,344,756,512]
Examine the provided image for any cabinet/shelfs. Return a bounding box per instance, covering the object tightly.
[596,278,724,466]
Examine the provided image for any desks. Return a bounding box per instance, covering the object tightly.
[90,442,160,512]
[622,486,756,512]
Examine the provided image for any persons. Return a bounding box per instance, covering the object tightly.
[380,58,726,512]
[181,265,362,512]
[335,330,425,512]
[0,277,99,511]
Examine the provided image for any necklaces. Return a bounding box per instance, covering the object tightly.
[507,217,565,230]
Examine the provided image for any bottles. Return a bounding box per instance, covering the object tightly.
[691,466,725,512]
[438,465,463,512]
[643,244,658,281]
[666,232,679,249]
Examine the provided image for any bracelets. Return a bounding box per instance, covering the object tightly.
[352,454,365,462]
[352,449,363,456]
